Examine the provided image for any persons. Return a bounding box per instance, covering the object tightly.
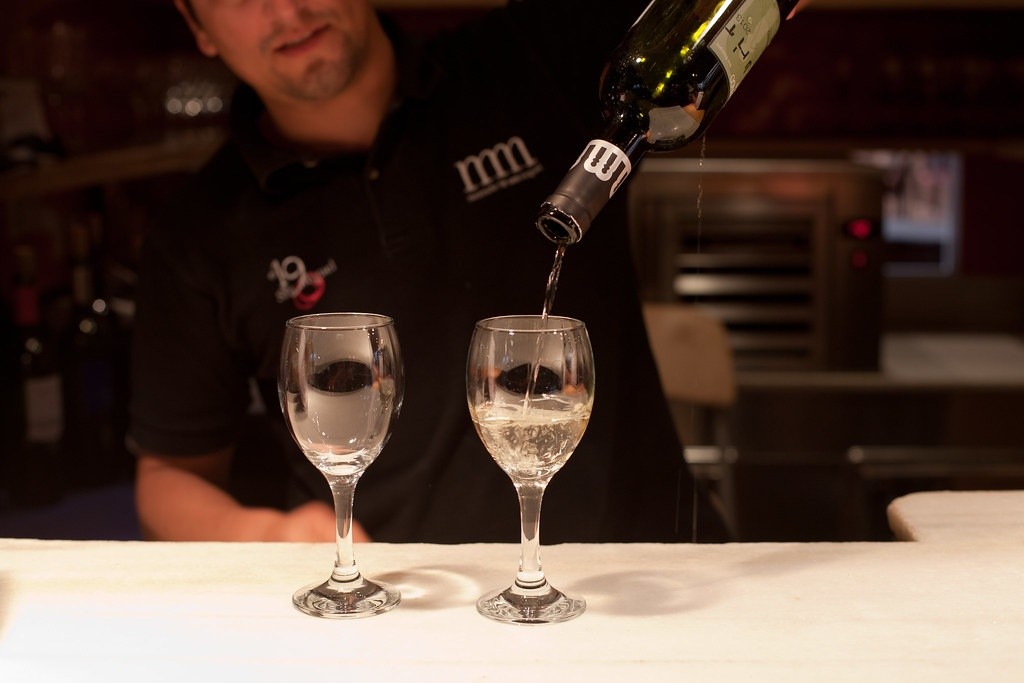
[126,0,812,544]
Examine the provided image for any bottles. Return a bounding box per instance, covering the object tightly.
[63,214,128,487]
[536,0,797,245]
[41,18,93,157]
[25,324,70,503]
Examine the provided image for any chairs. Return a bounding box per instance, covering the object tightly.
[641,304,735,525]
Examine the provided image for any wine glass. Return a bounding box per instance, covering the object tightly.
[465,314,595,623]
[277,312,407,621]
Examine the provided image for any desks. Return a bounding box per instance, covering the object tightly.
[0,542,1024,683]
